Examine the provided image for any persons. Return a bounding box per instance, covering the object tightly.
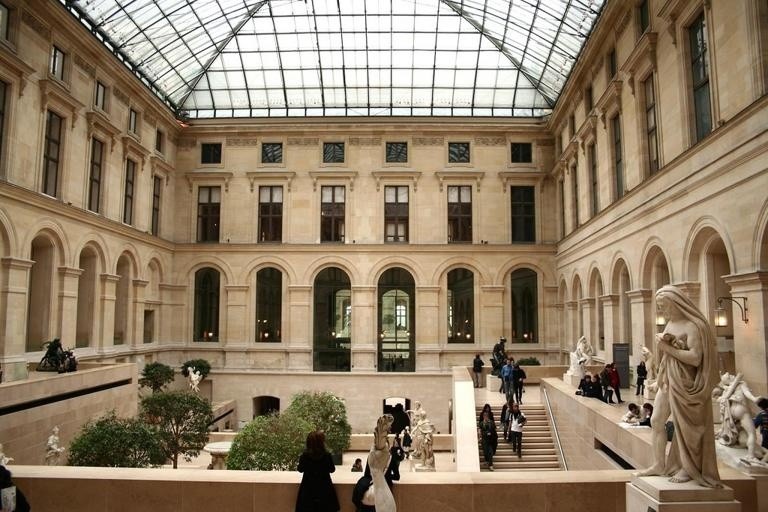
[350,458,364,472]
[633,283,725,489]
[187,366,203,392]
[45,426,65,465]
[0,442,14,465]
[473,337,526,471]
[0,466,31,512]
[712,371,768,469]
[637,342,656,380]
[296,430,339,512]
[406,399,435,468]
[576,335,595,365]
[577,361,653,429]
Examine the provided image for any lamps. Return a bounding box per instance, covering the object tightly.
[713,296,748,327]
[655,310,666,326]
